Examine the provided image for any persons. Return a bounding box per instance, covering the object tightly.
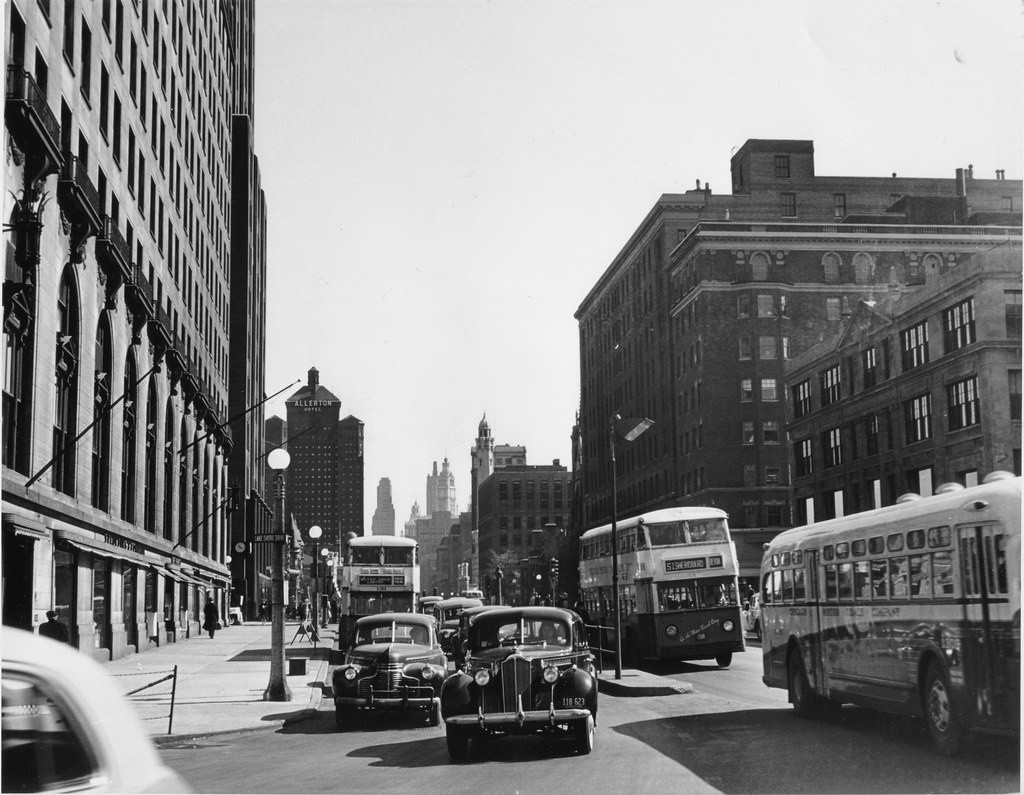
[541,621,559,644]
[338,605,341,618]
[701,522,725,541]
[286,604,306,620]
[261,605,267,625]
[446,609,460,620]
[40,611,69,643]
[202,597,219,639]
[410,627,425,644]
[704,586,727,606]
[656,528,677,545]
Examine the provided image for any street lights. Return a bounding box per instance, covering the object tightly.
[308,524,324,642]
[608,407,657,680]
[536,574,543,607]
[266,445,295,702]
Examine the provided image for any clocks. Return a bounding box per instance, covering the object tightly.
[235,541,246,553]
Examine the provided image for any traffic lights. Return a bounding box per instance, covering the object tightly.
[549,559,559,578]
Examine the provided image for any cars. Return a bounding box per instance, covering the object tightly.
[742,591,763,645]
[331,612,449,730]
[441,606,599,761]
[417,596,514,670]
[1,622,197,793]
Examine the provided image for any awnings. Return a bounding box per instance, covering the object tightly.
[73,541,222,588]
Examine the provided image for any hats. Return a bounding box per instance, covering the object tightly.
[208,597,213,601]
[46,610,58,617]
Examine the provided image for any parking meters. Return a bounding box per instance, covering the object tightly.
[320,547,333,628]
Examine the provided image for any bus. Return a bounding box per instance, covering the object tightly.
[578,506,747,668]
[754,470,1021,758]
[336,535,422,651]
